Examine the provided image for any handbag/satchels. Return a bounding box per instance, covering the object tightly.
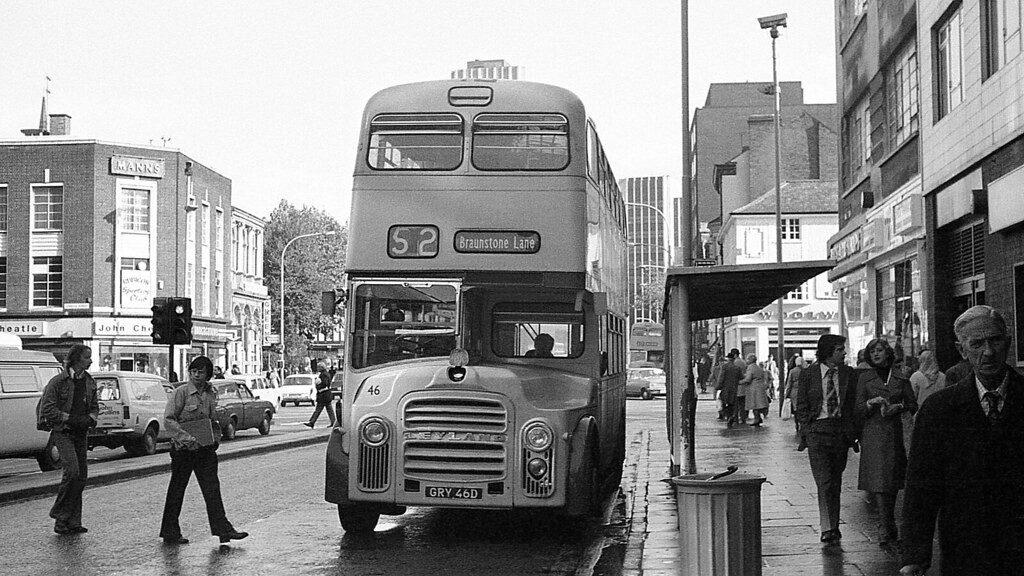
[781,397,791,421]
[35,397,54,431]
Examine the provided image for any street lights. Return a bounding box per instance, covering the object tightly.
[279,229,335,384]
[625,202,672,269]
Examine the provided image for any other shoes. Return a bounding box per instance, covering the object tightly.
[879,521,888,546]
[220,529,249,542]
[55,518,70,534]
[163,534,188,543]
[73,526,87,532]
[750,419,762,426]
[821,528,841,542]
[887,519,898,539]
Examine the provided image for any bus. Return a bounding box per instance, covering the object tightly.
[630,323,665,368]
[319,77,631,538]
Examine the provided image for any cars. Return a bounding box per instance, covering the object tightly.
[172,380,275,440]
[0,347,65,472]
[330,372,343,400]
[280,374,320,407]
[626,368,666,399]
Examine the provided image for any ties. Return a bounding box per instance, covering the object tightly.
[826,371,839,419]
[986,393,1001,426]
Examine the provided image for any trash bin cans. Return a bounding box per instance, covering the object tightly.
[672,473,767,576]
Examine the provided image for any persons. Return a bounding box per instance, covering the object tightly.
[854,349,874,379]
[269,367,281,383]
[945,342,972,387]
[896,305,1024,576]
[329,366,336,378]
[855,339,918,545]
[213,366,225,379]
[797,333,860,542]
[699,355,711,393]
[785,357,806,432]
[525,334,555,358]
[158,356,248,543]
[910,350,946,421]
[712,349,777,426]
[231,365,241,374]
[40,345,98,535]
[304,362,335,428]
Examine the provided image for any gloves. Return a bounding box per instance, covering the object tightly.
[65,414,92,430]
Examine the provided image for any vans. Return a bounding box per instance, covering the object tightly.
[87,371,177,456]
[232,375,281,413]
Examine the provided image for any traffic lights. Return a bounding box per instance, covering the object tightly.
[170,298,192,344]
[150,297,171,345]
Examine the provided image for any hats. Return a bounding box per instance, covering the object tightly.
[726,354,734,359]
